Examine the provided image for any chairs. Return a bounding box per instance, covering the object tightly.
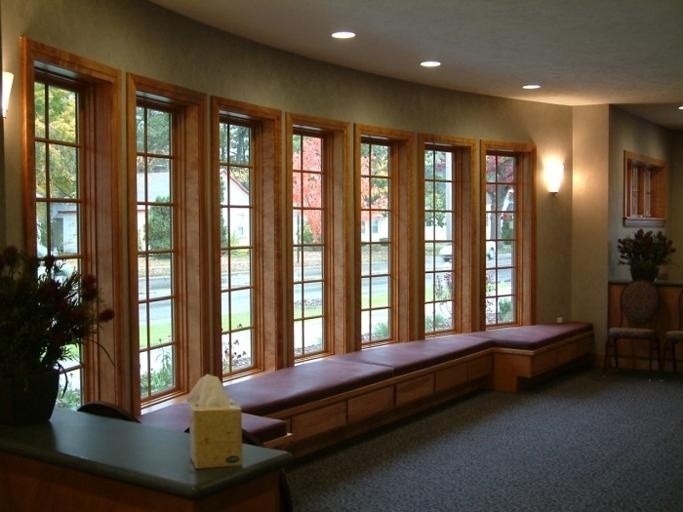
[603,279,682,374]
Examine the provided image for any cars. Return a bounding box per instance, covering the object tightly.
[438,239,498,264]
[36,244,78,291]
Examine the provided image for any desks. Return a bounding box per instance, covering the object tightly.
[0,405,290,512]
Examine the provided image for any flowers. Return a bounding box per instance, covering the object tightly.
[615,228,676,266]
[0,244,116,370]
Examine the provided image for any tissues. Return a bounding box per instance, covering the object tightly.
[184,374,242,471]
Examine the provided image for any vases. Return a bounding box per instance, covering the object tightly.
[629,267,659,280]
[0,371,60,425]
